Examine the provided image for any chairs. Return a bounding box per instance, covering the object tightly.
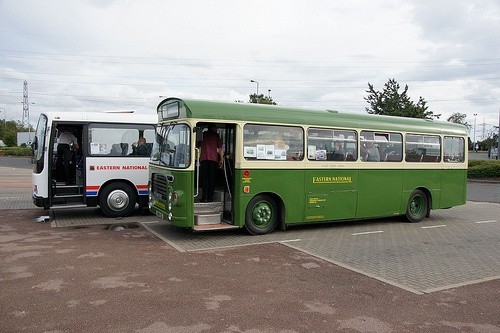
[327,147,439,162]
[111,143,128,156]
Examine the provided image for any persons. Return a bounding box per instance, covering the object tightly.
[55,129,79,185]
[129,138,147,157]
[197,123,223,202]
[330,142,345,161]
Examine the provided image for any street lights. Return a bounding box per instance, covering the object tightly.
[268,89,271,99]
[250,80,259,103]
[21,101,35,141]
[473,113,477,151]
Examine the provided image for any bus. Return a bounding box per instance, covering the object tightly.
[147,96,469,236]
[30,110,158,218]
